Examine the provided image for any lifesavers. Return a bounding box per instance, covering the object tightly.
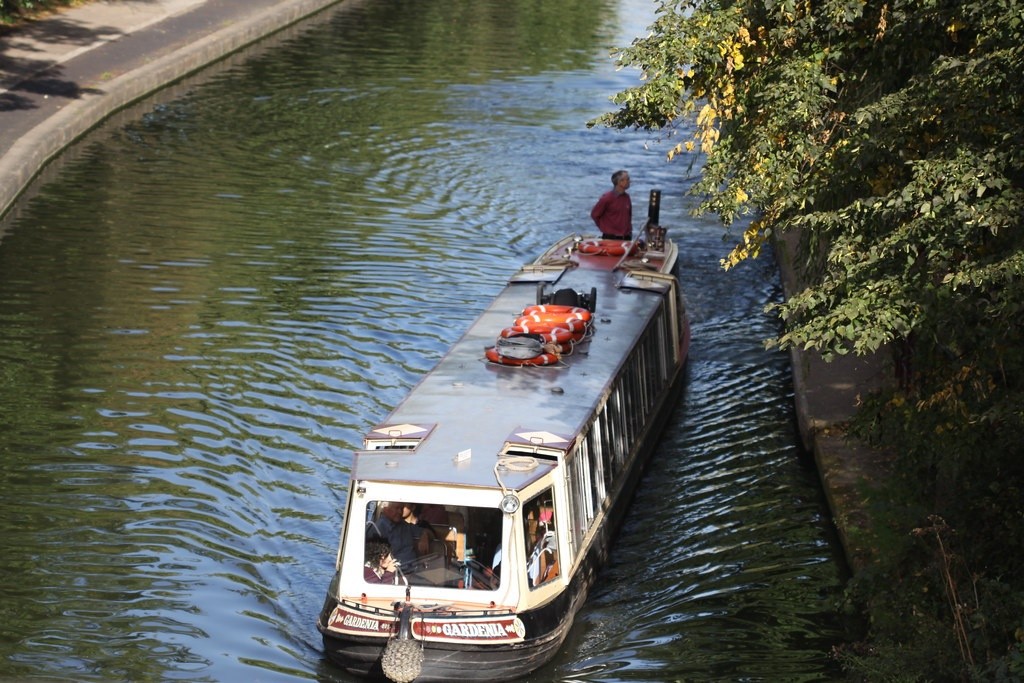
[485,303,592,367]
[578,239,640,255]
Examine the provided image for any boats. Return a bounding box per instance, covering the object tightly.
[315,189,692,683]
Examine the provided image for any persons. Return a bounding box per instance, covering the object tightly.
[590,169,632,240]
[365,494,559,590]
